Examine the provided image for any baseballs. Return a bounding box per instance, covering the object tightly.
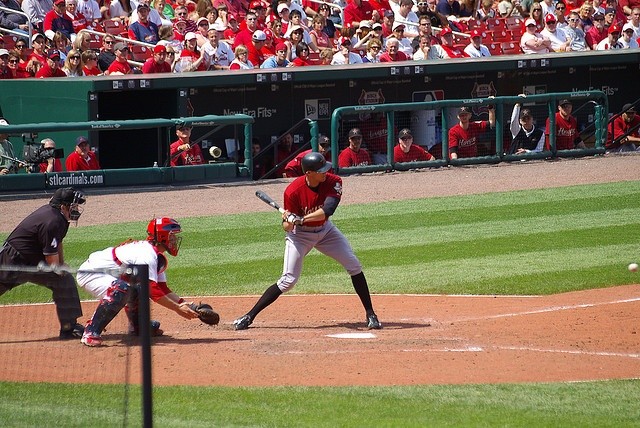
[628,263,638,272]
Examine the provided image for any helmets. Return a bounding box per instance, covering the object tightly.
[148,218,182,256]
[53,188,86,228]
[302,152,332,172]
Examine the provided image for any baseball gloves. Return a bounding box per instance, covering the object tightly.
[191,304,220,324]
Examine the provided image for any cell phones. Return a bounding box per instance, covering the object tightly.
[343,47,350,53]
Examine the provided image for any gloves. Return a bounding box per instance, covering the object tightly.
[286,213,304,225]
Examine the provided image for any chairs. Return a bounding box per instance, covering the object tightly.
[452,17,530,55]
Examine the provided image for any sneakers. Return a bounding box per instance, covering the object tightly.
[234,315,253,330]
[60,323,85,339]
[81,332,101,347]
[368,315,381,329]
[129,329,164,337]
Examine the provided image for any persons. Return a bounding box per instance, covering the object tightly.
[233,152,381,331]
[251,138,265,181]
[606,104,640,153]
[0,118,26,174]
[282,134,331,178]
[0,188,86,339]
[75,216,201,346]
[506,93,545,155]
[359,113,398,153]
[270,130,301,179]
[1,1,640,79]
[394,128,435,162]
[169,122,204,166]
[448,96,496,160]
[38,137,63,173]
[65,136,101,171]
[338,128,372,166]
[545,99,587,150]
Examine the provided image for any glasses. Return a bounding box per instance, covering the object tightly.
[556,7,565,10]
[585,9,590,12]
[370,47,381,50]
[51,58,60,61]
[528,25,535,28]
[248,18,258,20]
[178,13,186,15]
[533,9,542,12]
[69,4,75,5]
[106,41,114,44]
[396,30,404,32]
[624,13,630,16]
[420,24,430,26]
[568,19,580,22]
[320,8,329,11]
[276,25,281,27]
[70,56,80,60]
[417,2,427,6]
[293,32,302,35]
[301,49,308,52]
[240,52,247,55]
[166,52,173,55]
[15,45,25,50]
[547,21,555,25]
[10,60,17,63]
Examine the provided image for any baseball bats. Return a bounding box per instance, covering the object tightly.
[255,189,300,225]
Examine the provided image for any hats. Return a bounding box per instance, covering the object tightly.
[56,0,64,5]
[197,17,208,25]
[48,51,60,59]
[252,30,266,40]
[392,21,405,31]
[277,3,289,13]
[41,139,55,148]
[137,4,148,12]
[525,19,536,26]
[399,129,411,138]
[288,25,304,36]
[470,30,481,38]
[520,109,531,119]
[623,103,637,113]
[360,20,370,28]
[176,122,192,130]
[276,43,287,50]
[175,18,186,25]
[250,1,263,9]
[261,2,267,9]
[318,136,330,144]
[340,36,350,44]
[228,15,236,22]
[559,100,571,105]
[605,8,616,15]
[623,24,634,31]
[0,119,9,126]
[385,10,393,18]
[0,49,9,56]
[594,12,604,18]
[609,25,620,33]
[76,137,88,146]
[154,45,166,53]
[349,128,363,138]
[32,33,45,41]
[545,14,556,23]
[441,28,452,35]
[114,43,128,50]
[372,23,382,29]
[185,32,197,40]
[458,106,470,114]
